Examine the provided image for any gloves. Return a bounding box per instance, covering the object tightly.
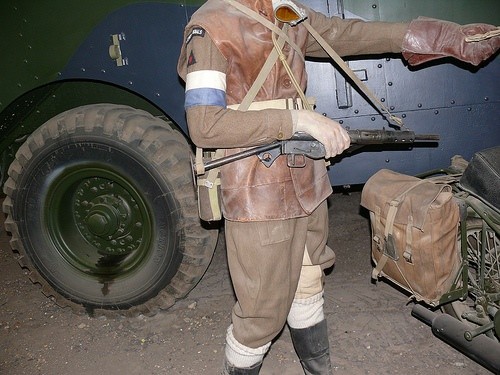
[401,16,499,66]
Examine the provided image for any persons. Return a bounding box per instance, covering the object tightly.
[176,0,497,375]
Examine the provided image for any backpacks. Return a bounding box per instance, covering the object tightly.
[360,168,464,306]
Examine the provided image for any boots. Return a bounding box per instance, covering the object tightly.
[225,361,262,375]
[289,318,333,375]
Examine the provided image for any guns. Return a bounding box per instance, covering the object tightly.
[193,130,442,176]
